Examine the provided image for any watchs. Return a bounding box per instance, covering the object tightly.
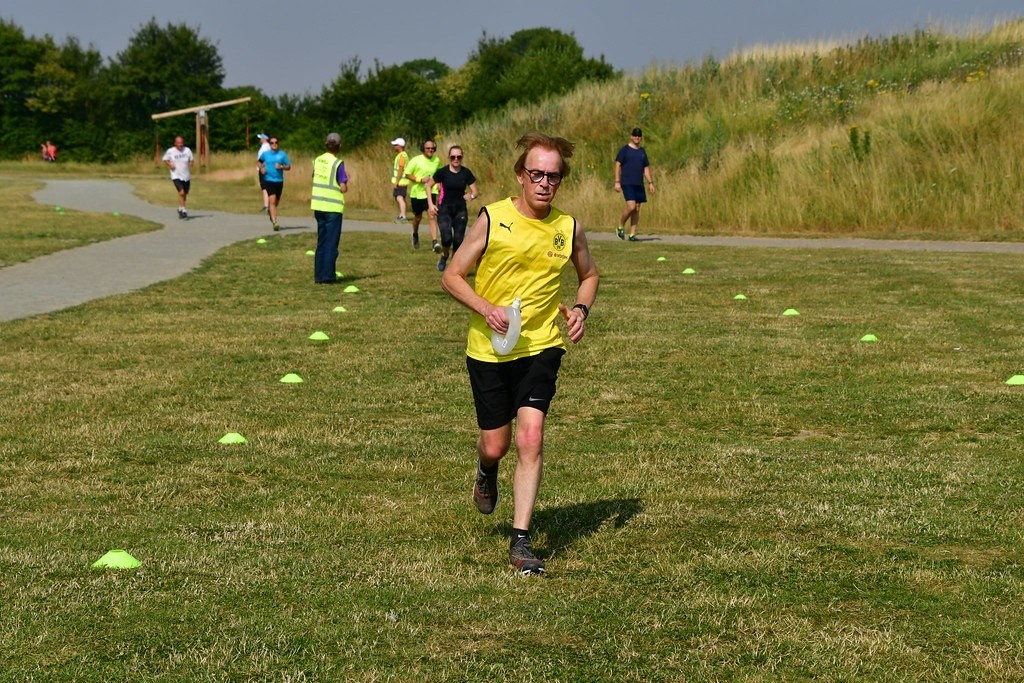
[573,304,588,322]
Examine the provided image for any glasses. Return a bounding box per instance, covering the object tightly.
[449,156,463,159]
[425,147,434,150]
[270,142,278,144]
[523,167,563,186]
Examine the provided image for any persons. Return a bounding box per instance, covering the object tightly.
[40,142,48,160]
[162,135,194,218]
[310,133,348,283]
[47,140,57,162]
[426,145,479,271]
[441,133,599,575]
[257,135,291,231]
[405,139,444,252]
[257,133,271,213]
[614,127,655,241]
[391,138,410,224]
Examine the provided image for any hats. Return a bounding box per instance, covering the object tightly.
[632,128,643,136]
[391,138,405,147]
[325,133,340,145]
[257,134,268,139]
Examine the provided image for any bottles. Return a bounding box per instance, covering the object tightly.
[425,174,431,187]
[491,298,521,355]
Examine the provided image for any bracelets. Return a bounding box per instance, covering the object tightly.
[421,179,422,182]
[416,177,421,182]
[281,164,284,168]
[615,181,620,183]
[471,194,474,199]
[649,181,653,184]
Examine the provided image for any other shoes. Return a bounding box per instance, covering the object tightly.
[413,233,418,245]
[274,223,280,231]
[432,243,442,253]
[182,212,187,218]
[397,215,406,220]
[178,209,183,218]
[438,255,448,271]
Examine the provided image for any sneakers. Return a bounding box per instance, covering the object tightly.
[616,227,625,240]
[508,538,545,574]
[473,459,498,515]
[629,234,639,241]
